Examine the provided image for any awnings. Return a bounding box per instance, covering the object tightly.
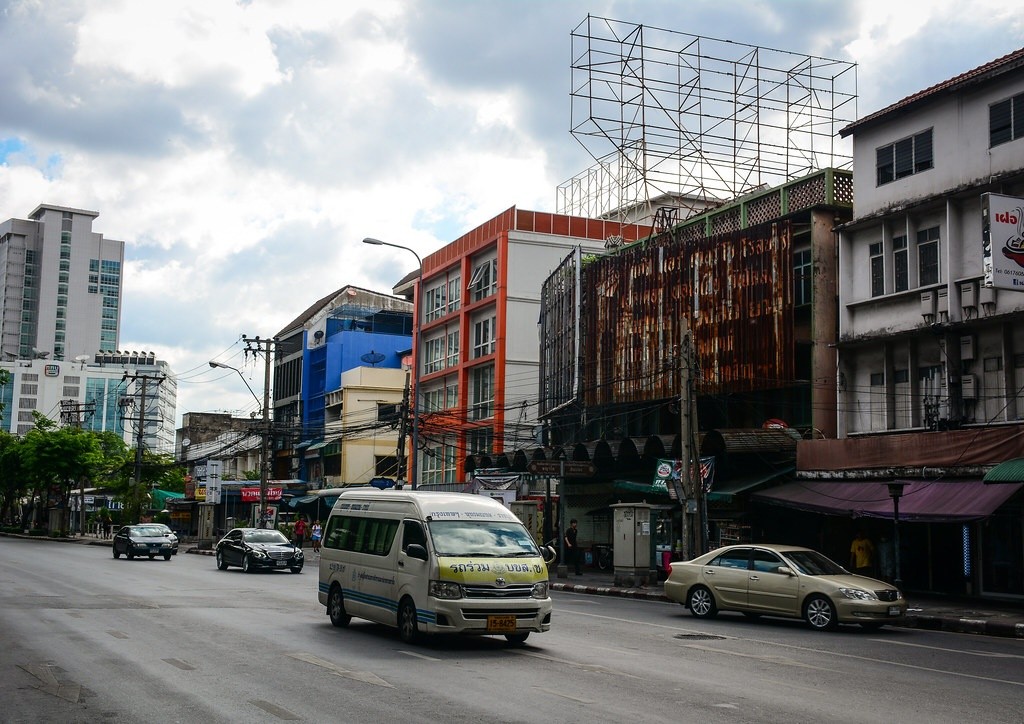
[751,479,1024,527]
[306,439,334,451]
[614,464,796,503]
[294,438,320,449]
[983,457,1024,485]
[281,495,319,508]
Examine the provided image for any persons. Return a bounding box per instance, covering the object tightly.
[565,519,583,575]
[850,528,876,574]
[293,515,323,552]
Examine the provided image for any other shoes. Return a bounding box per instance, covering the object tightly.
[576,572,582,575]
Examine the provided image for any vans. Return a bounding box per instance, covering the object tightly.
[318,488,553,644]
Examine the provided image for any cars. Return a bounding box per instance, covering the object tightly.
[136,523,179,555]
[112,525,172,560]
[664,543,907,632]
[216,528,304,574]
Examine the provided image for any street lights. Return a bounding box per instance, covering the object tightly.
[209,360,269,530]
[362,237,423,491]
